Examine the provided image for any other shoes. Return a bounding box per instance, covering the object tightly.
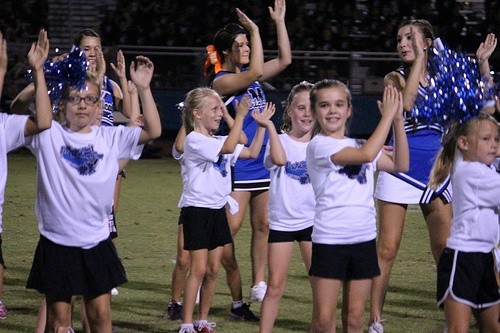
[110,288,119,295]
[368,317,387,333]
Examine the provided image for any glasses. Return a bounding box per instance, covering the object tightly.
[64,96,99,106]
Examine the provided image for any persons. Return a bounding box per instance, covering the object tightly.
[0,29,162,333]
[171,87,275,333]
[306,79,409,333]
[426,113,500,333]
[194,0,292,304]
[367,19,500,333]
[0,0,49,115]
[251,81,318,333]
[99,0,500,90]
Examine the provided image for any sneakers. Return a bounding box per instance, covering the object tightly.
[0,302,7,319]
[250,282,267,302]
[194,320,216,333]
[178,323,197,333]
[168,299,184,322]
[230,302,260,322]
